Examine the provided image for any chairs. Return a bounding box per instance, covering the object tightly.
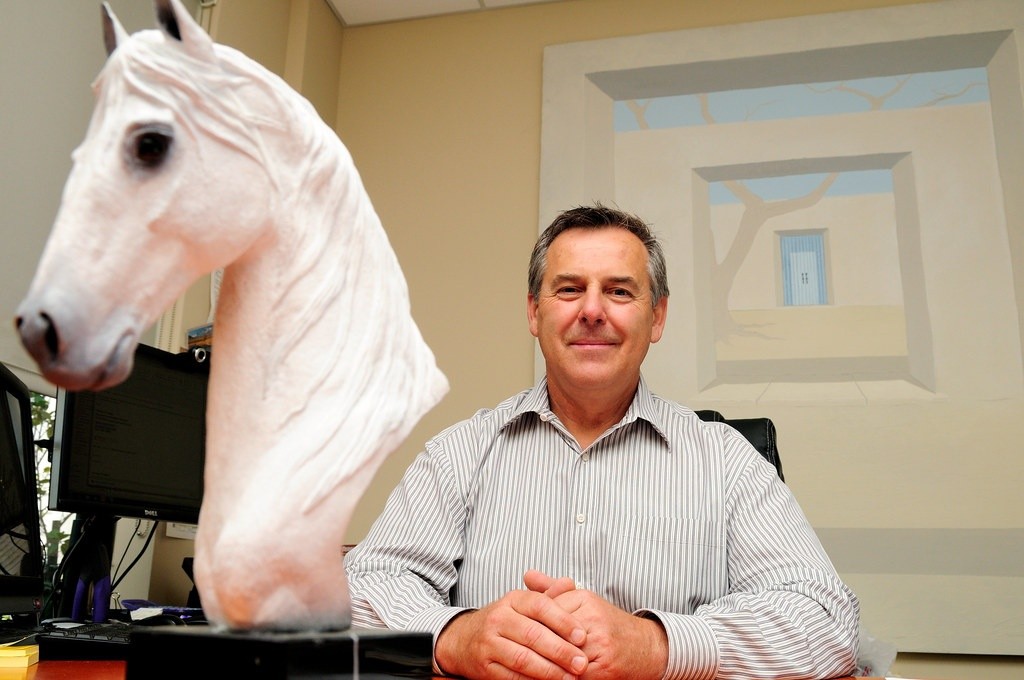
[693,409,785,482]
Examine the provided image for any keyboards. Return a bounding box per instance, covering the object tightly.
[34,622,130,658]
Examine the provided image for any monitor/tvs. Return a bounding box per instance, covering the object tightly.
[49,342,210,624]
[0,361,46,615]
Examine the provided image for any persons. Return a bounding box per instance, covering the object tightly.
[341,197,863,680]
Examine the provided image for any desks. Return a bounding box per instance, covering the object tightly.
[0,660,126,680]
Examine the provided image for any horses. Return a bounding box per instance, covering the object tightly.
[12,0,451,634]
